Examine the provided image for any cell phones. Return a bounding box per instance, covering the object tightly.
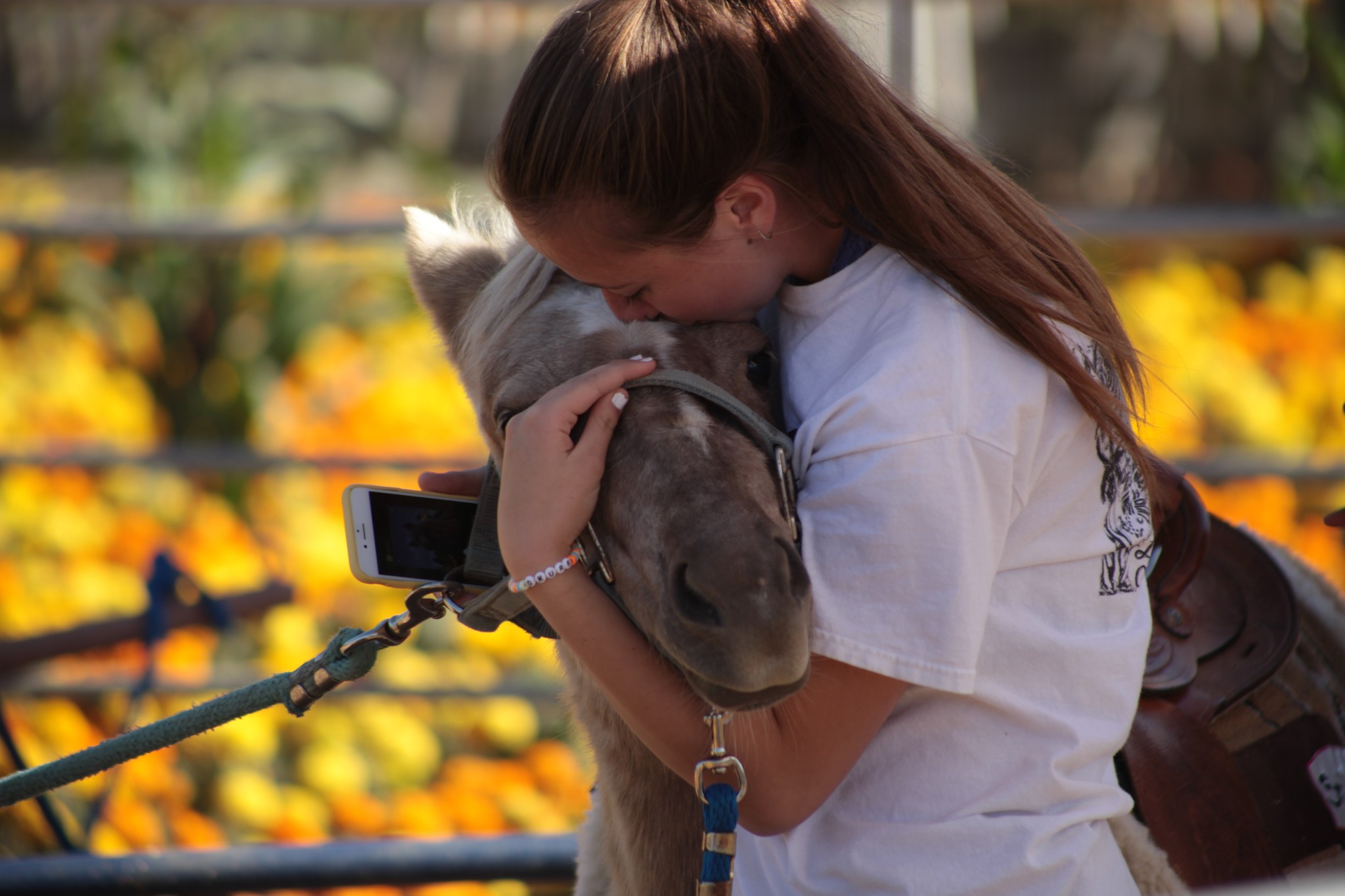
[343,482,511,597]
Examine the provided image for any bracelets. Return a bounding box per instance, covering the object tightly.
[505,541,582,593]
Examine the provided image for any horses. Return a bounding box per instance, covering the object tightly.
[401,179,1345,896]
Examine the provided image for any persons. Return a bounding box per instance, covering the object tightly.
[406,0,1158,896]
